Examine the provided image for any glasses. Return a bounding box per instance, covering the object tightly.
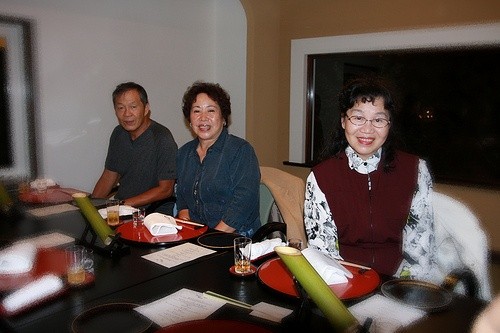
[343,112,391,128]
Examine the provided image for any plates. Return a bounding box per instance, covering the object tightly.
[197,232,246,248]
[256,257,381,302]
[71,302,153,333]
[115,218,208,244]
[380,278,453,309]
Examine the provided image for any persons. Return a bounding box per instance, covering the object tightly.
[177,82,261,238]
[91,83,178,217]
[304,79,434,282]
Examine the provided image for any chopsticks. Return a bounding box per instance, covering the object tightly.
[338,261,372,270]
[203,291,253,310]
[174,219,204,227]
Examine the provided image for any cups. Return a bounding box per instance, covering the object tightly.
[17,174,29,194]
[106,199,120,226]
[131,208,145,226]
[234,237,252,274]
[36,178,47,194]
[65,245,85,286]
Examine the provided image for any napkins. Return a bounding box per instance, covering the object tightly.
[302,245,354,285]
[31,177,57,191]
[347,293,427,333]
[143,212,183,236]
[239,237,288,261]
[0,243,37,274]
[98,205,141,220]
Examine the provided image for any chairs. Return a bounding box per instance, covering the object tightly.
[432,191,493,302]
[259,164,307,251]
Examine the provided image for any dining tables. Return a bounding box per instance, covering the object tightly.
[0,190,257,333]
[7,240,487,333]
